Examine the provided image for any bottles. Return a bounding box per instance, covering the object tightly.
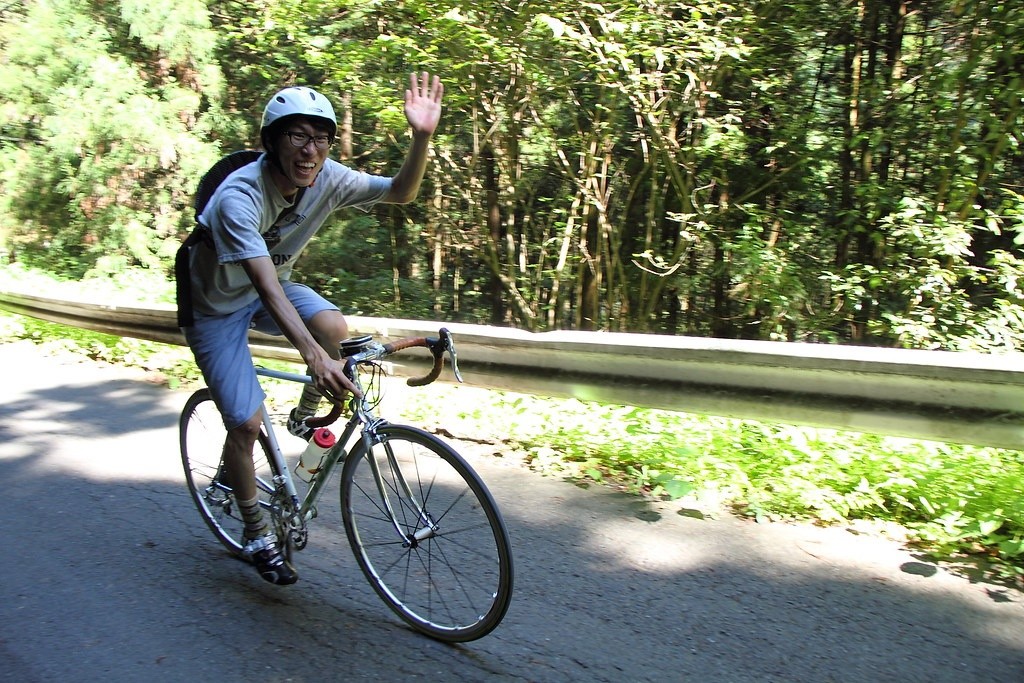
[295,429,335,482]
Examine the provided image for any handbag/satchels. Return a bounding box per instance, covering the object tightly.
[194,150,264,224]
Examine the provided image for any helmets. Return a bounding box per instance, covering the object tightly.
[259,86,338,153]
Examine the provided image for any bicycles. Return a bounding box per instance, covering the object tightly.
[177,317,516,644]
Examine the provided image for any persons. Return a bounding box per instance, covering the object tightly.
[175,72,445,585]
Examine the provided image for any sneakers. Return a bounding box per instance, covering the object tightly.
[287,407,347,465]
[241,529,299,586]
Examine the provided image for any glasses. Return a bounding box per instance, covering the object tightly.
[281,130,335,150]
